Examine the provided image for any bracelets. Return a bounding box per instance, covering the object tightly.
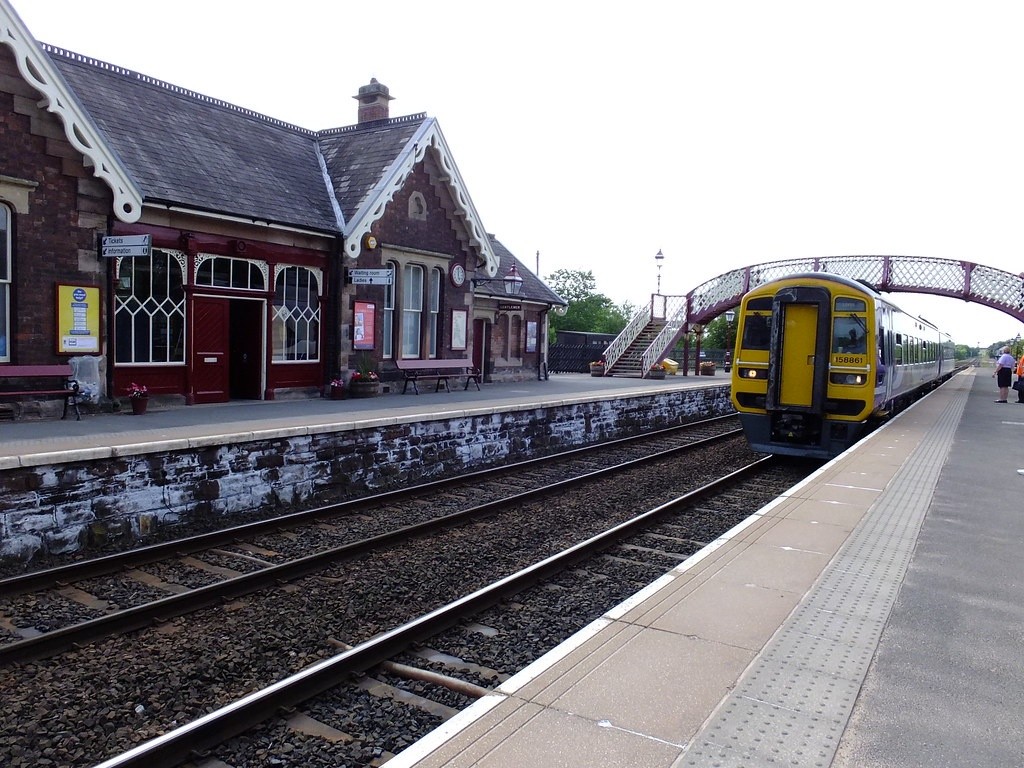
[994,372,996,373]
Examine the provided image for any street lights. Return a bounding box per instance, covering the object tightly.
[654,248,665,295]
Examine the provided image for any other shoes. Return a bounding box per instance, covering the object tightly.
[994,399,1007,403]
[1015,400,1024,403]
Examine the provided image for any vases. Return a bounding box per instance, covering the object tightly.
[649,369,665,378]
[701,367,715,376]
[331,391,344,400]
[590,366,604,376]
[351,381,378,397]
[132,397,149,415]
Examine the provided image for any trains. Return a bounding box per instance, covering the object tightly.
[730,270,956,460]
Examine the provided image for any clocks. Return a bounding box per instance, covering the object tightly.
[450,262,465,288]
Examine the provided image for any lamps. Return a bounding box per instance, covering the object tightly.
[471,261,523,295]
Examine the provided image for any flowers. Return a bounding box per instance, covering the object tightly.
[329,375,344,391]
[699,360,714,367]
[588,361,604,366]
[651,363,664,370]
[350,353,378,383]
[127,382,148,399]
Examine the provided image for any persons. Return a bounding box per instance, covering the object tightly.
[992,346,1015,403]
[1015,346,1024,403]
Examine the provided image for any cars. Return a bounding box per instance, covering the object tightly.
[699,352,707,358]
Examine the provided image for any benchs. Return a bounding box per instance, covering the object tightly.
[394,360,481,394]
[0,365,81,421]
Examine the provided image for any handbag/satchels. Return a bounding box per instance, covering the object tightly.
[1012,381,1024,391]
